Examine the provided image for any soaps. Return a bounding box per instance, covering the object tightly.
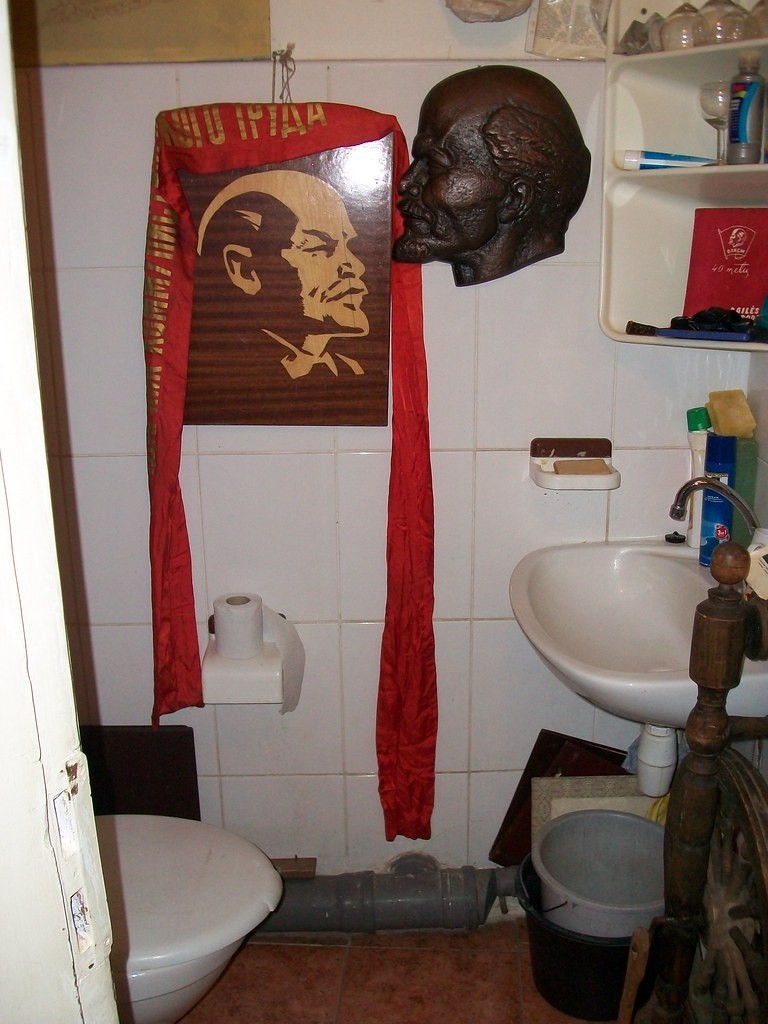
[553,458,611,481]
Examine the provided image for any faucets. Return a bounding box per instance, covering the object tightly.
[669,477,764,548]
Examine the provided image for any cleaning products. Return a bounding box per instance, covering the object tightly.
[685,407,716,549]
[696,429,738,569]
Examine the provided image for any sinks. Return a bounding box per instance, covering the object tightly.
[508,531,768,728]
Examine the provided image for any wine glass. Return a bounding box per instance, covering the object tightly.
[697,79,734,169]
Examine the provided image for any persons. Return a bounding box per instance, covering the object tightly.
[391,64,590,288]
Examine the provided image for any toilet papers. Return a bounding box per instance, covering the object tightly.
[210,591,308,719]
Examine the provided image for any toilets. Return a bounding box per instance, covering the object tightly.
[91,790,288,1024]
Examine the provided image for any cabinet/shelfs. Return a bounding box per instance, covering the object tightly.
[599,0,768,353]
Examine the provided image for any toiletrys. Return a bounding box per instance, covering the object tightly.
[743,527,768,557]
[618,149,724,173]
